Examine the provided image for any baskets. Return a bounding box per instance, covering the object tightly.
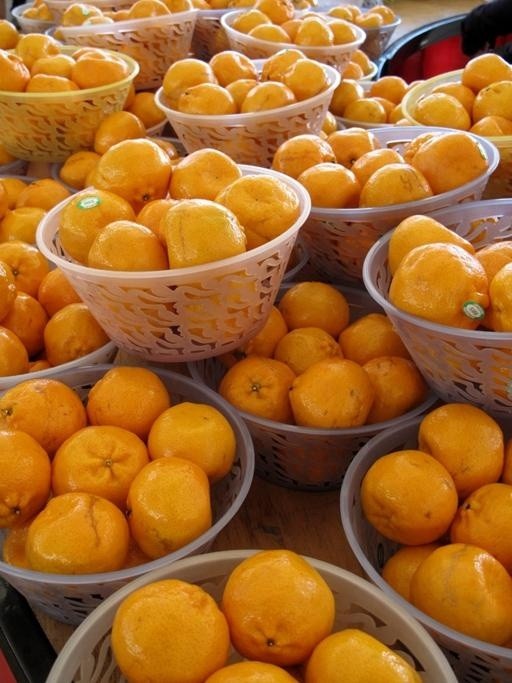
[0,0,512,683]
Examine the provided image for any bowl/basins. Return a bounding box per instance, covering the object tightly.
[371,8,509,84]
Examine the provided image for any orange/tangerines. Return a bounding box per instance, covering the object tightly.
[0,0,512,683]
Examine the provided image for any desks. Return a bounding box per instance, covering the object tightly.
[0,0,509,682]
